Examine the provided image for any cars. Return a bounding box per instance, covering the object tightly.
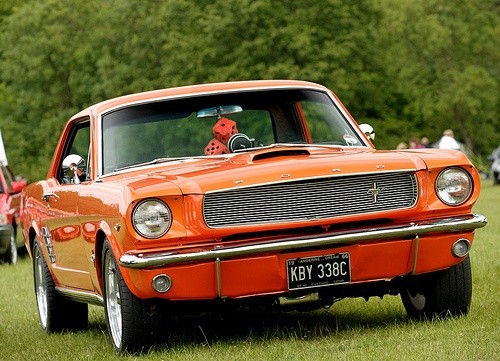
[0,160,30,264]
[20,79,489,357]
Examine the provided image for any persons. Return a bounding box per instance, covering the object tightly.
[410,138,422,149]
[433,129,466,156]
[420,133,433,148]
